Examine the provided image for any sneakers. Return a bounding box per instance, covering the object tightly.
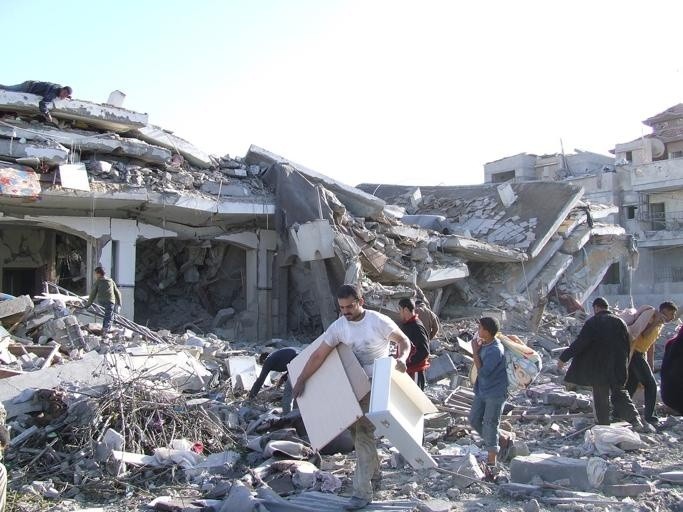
[631,417,644,432]
[646,416,658,422]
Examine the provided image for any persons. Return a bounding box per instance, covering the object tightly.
[292,284,411,511]
[0,80,72,122]
[468,317,509,469]
[396,299,430,446]
[249,347,308,416]
[661,326,682,415]
[85,267,122,339]
[611,301,679,423]
[559,298,644,431]
[414,301,439,391]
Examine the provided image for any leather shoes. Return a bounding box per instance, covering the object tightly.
[371,478,381,491]
[343,496,372,510]
